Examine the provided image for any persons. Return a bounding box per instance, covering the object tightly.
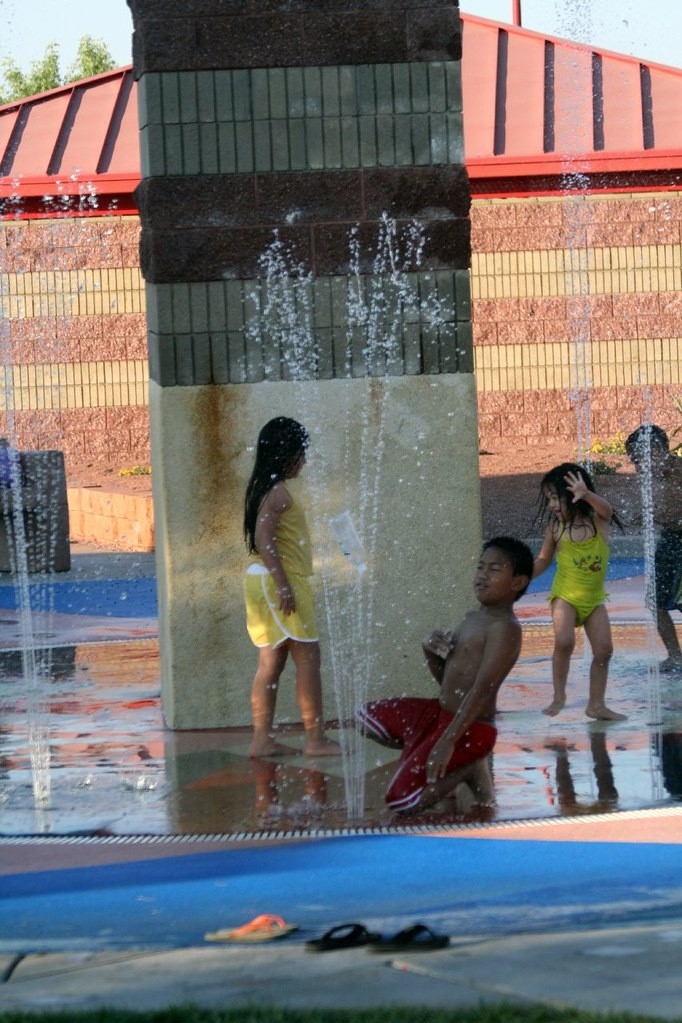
[251,762,329,832]
[625,422,682,674]
[241,416,356,760]
[359,535,536,815]
[544,723,619,816]
[530,462,628,723]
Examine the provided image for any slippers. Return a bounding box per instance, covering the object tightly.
[203,913,298,941]
[366,922,451,952]
[306,924,382,952]
